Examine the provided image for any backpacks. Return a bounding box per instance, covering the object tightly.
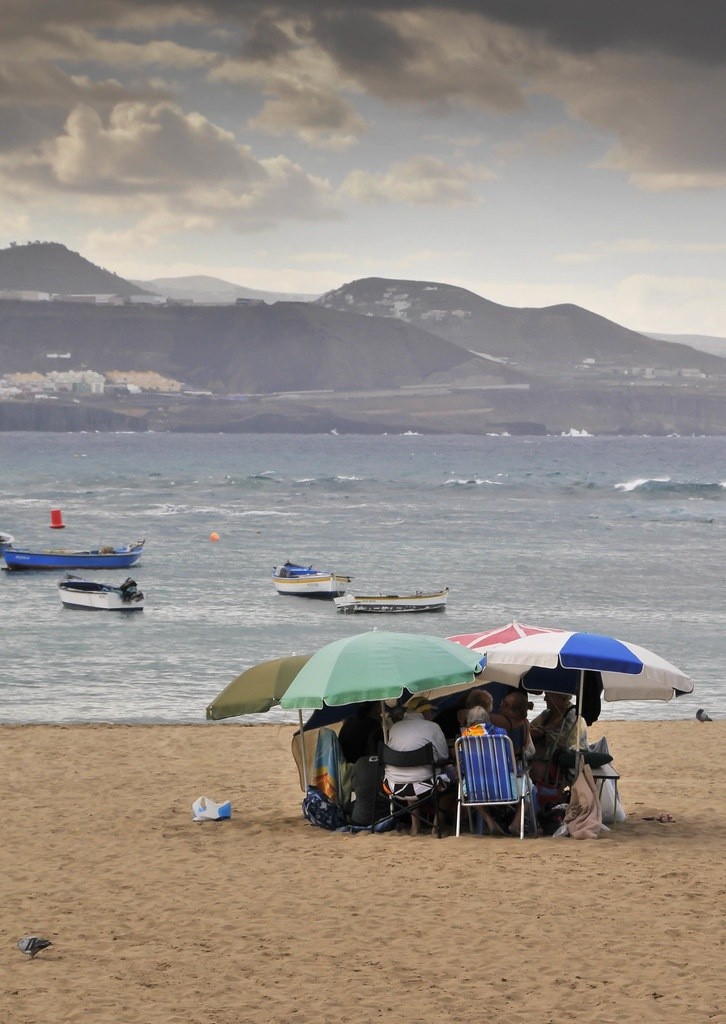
[302,785,337,829]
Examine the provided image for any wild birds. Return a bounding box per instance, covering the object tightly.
[696,708,712,722]
[17,935,53,960]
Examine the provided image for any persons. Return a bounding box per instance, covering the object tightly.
[337,689,588,835]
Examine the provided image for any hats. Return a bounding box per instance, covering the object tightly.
[406,696,438,714]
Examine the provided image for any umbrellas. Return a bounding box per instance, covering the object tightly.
[475,631,695,776]
[280,628,486,744]
[445,618,561,654]
[207,654,312,798]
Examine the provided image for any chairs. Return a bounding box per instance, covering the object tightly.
[371,738,449,839]
[452,733,538,838]
[291,726,356,819]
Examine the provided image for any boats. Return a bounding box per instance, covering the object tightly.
[271,560,350,599]
[57,572,147,613]
[0,540,146,569]
[0,532,14,552]
[333,587,450,614]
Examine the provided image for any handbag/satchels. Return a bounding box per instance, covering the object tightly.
[596,777,626,823]
[564,757,603,839]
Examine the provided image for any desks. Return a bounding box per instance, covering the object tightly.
[568,759,621,824]
